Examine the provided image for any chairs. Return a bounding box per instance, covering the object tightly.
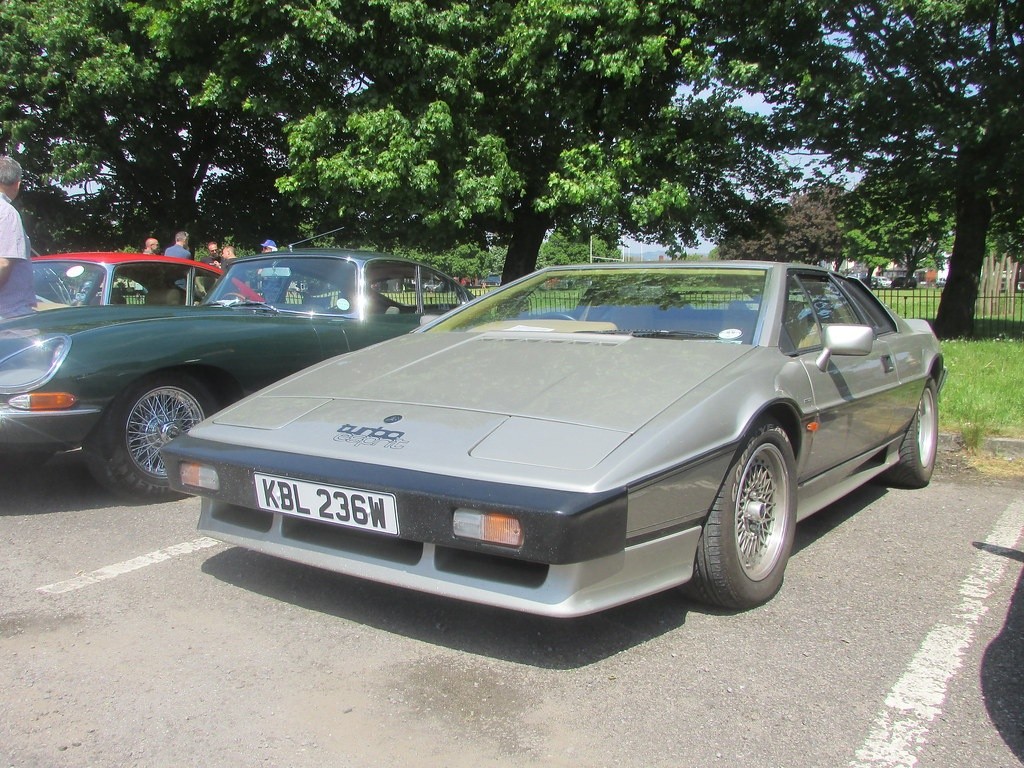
[90,293,125,304]
[143,289,185,304]
[785,298,814,350]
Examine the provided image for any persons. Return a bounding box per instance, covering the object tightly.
[200,242,222,268]
[142,238,159,255]
[164,231,191,260]
[0,150,33,296]
[214,245,238,272]
[261,240,278,253]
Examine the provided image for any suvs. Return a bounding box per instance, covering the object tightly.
[892,277,916,288]
[873,277,893,286]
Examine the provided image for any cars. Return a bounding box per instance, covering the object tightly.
[160,261,948,620]
[0,248,477,502]
[28,254,262,329]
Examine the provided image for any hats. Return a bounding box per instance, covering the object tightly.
[261,240,276,248]
